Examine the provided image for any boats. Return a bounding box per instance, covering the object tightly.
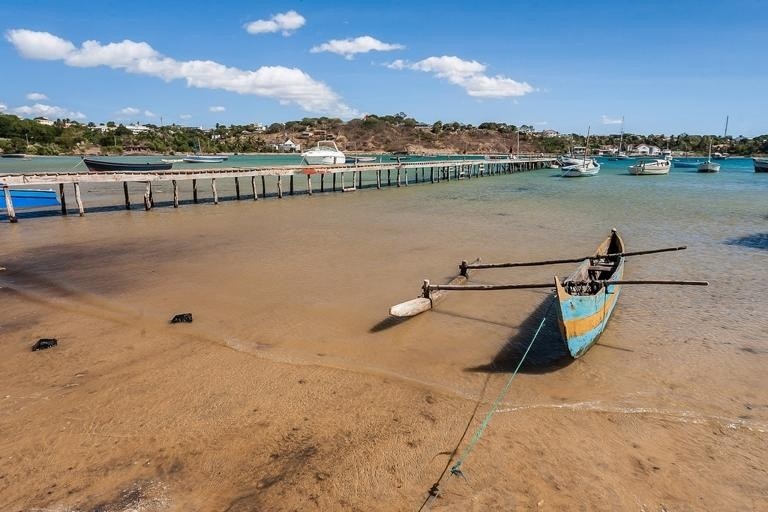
[560,158,600,177]
[81,154,173,171]
[697,140,720,173]
[554,227,625,360]
[183,156,229,163]
[627,159,671,175]
[753,158,768,173]
[608,150,629,161]
[300,141,377,165]
